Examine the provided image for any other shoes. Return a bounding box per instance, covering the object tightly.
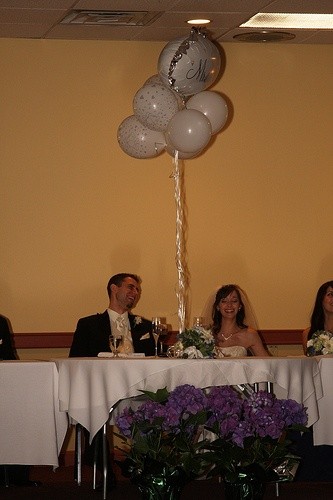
[106,473,115,487]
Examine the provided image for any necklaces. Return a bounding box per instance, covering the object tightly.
[222,333,232,340]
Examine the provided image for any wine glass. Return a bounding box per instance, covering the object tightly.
[152,317,166,359]
[109,335,124,358]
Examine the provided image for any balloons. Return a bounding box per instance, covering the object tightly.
[117,115,164,159]
[133,83,179,131]
[158,26,221,97]
[167,109,212,152]
[165,145,199,159]
[144,73,184,111]
[185,91,228,136]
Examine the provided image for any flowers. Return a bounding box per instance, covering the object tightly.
[174,323,219,357]
[305,331,333,355]
[113,381,310,500]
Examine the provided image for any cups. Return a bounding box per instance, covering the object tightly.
[194,317,206,329]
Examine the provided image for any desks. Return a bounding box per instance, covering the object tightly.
[0,351,333,500]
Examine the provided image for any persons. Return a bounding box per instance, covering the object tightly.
[302,281,333,355]
[0,315,17,360]
[69,273,160,357]
[201,284,269,357]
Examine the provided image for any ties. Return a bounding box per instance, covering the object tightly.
[116,317,125,353]
